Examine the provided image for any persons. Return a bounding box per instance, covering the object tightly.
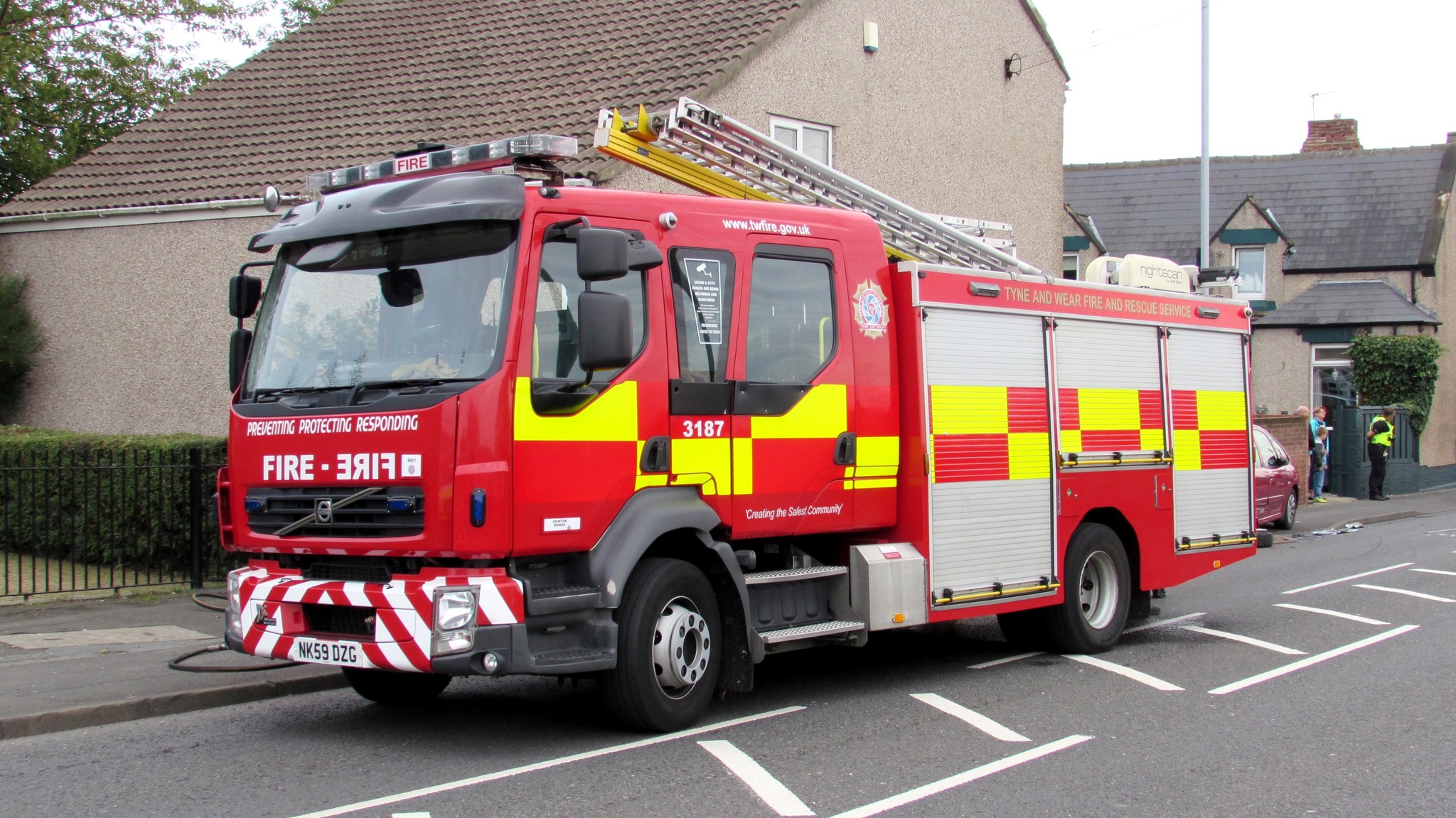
[1293,406,1315,500]
[1310,406,1327,465]
[1314,425,1328,503]
[1367,408,1394,501]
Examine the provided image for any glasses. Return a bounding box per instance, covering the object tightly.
[1319,411,1326,414]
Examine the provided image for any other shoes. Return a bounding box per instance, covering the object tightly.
[1308,496,1313,500]
[1380,494,1390,500]
[1322,497,1326,500]
[1369,495,1386,501]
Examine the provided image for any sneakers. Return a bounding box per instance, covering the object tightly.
[1313,497,1327,503]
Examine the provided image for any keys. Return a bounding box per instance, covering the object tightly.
[1383,450,1388,462]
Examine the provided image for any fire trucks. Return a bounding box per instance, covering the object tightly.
[213,93,1261,733]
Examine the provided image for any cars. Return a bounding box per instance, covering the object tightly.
[1254,425,1299,528]
